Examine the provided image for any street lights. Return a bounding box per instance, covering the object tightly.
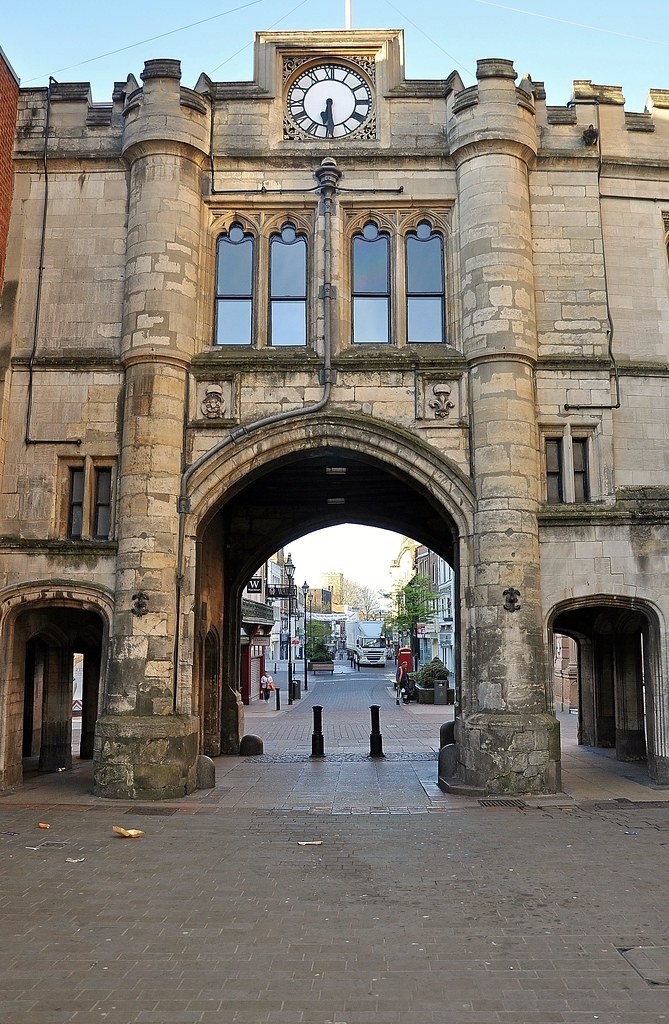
[307,592,314,662]
[283,552,296,706]
[301,581,309,691]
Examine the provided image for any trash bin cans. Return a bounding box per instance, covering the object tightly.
[434,680,448,705]
[292,679,301,699]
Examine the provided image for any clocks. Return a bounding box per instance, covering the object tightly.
[286,63,370,139]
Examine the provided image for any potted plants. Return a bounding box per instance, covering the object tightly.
[307,621,333,671]
[414,657,455,704]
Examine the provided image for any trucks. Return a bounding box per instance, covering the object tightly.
[344,619,387,668]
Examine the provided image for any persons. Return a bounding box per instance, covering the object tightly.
[396,661,411,703]
[261,671,273,704]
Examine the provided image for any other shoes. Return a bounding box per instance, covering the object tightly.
[264,700,270,704]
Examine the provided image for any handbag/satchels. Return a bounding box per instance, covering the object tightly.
[266,682,275,691]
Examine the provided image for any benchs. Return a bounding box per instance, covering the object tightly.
[311,663,335,676]
